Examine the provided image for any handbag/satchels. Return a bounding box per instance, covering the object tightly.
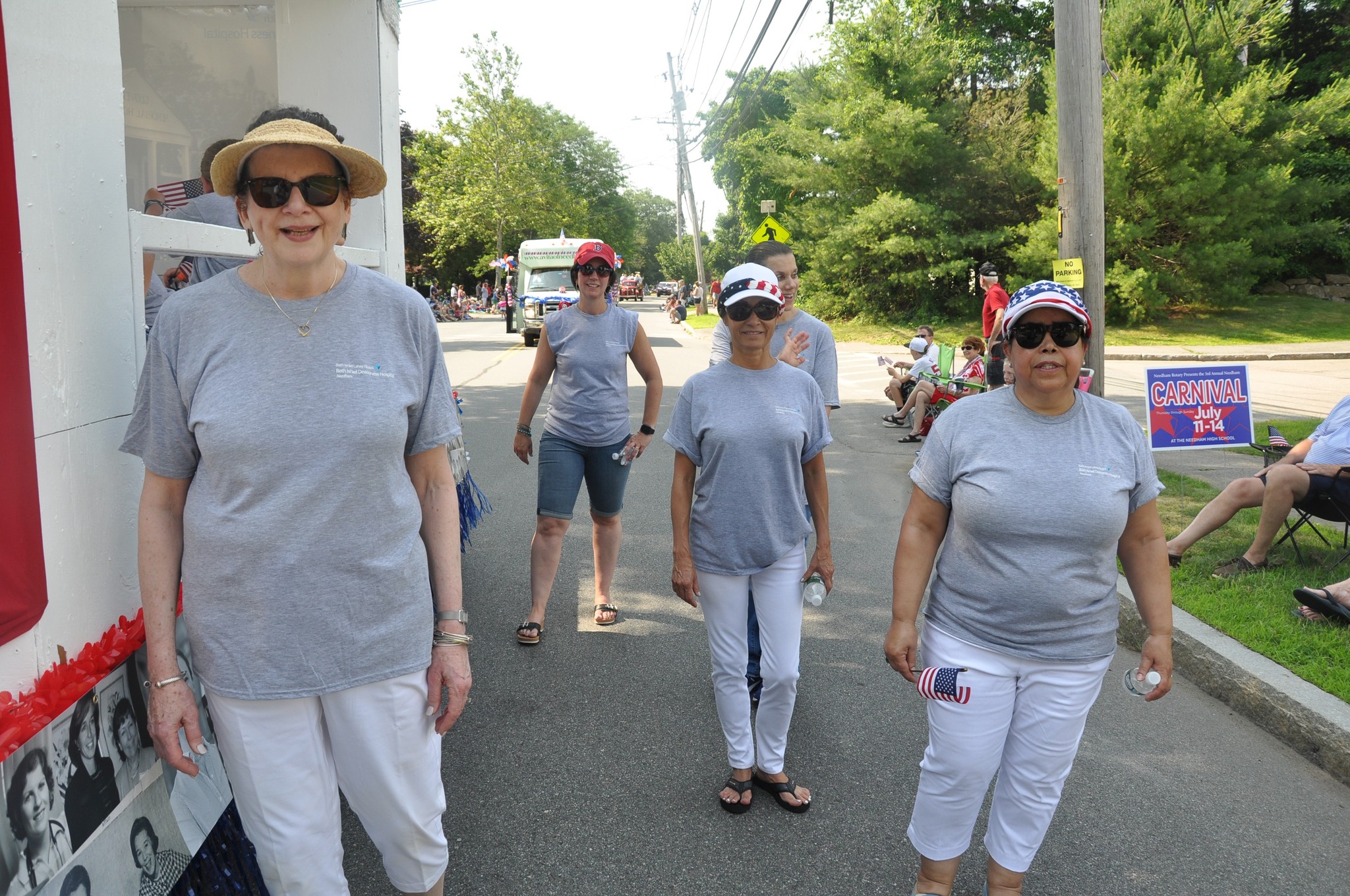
[920,417,933,436]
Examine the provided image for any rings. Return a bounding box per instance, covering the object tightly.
[635,447,639,449]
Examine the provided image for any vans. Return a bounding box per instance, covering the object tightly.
[657,282,680,298]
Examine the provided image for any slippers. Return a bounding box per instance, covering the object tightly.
[515,617,546,644]
[1291,607,1332,623]
[1293,586,1350,625]
[753,770,811,814]
[719,772,755,814]
[594,603,618,625]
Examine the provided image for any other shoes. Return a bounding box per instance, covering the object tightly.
[745,674,764,702]
[883,416,910,428]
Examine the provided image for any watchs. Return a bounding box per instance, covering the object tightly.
[436,608,469,624]
[640,424,655,435]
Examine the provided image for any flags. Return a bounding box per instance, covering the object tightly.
[917,668,970,704]
[155,176,206,212]
[1267,425,1290,447]
[173,255,194,281]
[489,257,502,267]
[884,356,893,365]
[878,356,886,366]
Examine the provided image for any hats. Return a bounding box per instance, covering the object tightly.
[1002,280,1089,334]
[574,242,616,269]
[719,263,783,307]
[976,263,998,277]
[903,337,928,352]
[210,119,388,200]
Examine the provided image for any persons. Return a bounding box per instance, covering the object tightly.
[1166,395,1350,628]
[883,283,1173,896]
[134,139,255,347]
[512,242,664,646]
[423,279,508,323]
[5,650,237,896]
[665,262,834,811]
[663,278,722,324]
[127,109,473,896]
[710,239,840,721]
[532,269,545,284]
[882,261,1015,456]
[619,274,641,286]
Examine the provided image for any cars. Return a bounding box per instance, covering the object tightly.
[618,279,644,302]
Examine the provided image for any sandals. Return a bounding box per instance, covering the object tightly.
[881,413,905,426]
[898,432,922,442]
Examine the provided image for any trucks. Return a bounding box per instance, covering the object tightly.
[503,237,605,347]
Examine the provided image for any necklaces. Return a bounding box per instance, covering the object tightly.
[255,265,339,338]
[194,754,201,763]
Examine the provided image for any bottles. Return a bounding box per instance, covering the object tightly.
[803,571,827,608]
[1122,668,1161,697]
[613,446,638,465]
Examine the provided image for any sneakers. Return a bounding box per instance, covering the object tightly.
[1212,557,1269,581]
[1168,553,1182,570]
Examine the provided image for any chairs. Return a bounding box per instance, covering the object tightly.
[899,343,988,429]
[1250,441,1350,580]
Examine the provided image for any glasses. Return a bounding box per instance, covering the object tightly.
[727,299,779,321]
[1010,321,1085,348]
[915,334,926,338]
[576,263,612,278]
[244,174,347,210]
[961,346,972,350]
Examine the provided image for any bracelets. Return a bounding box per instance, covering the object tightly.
[432,627,474,646]
[143,670,188,689]
[953,390,957,396]
[516,423,532,437]
